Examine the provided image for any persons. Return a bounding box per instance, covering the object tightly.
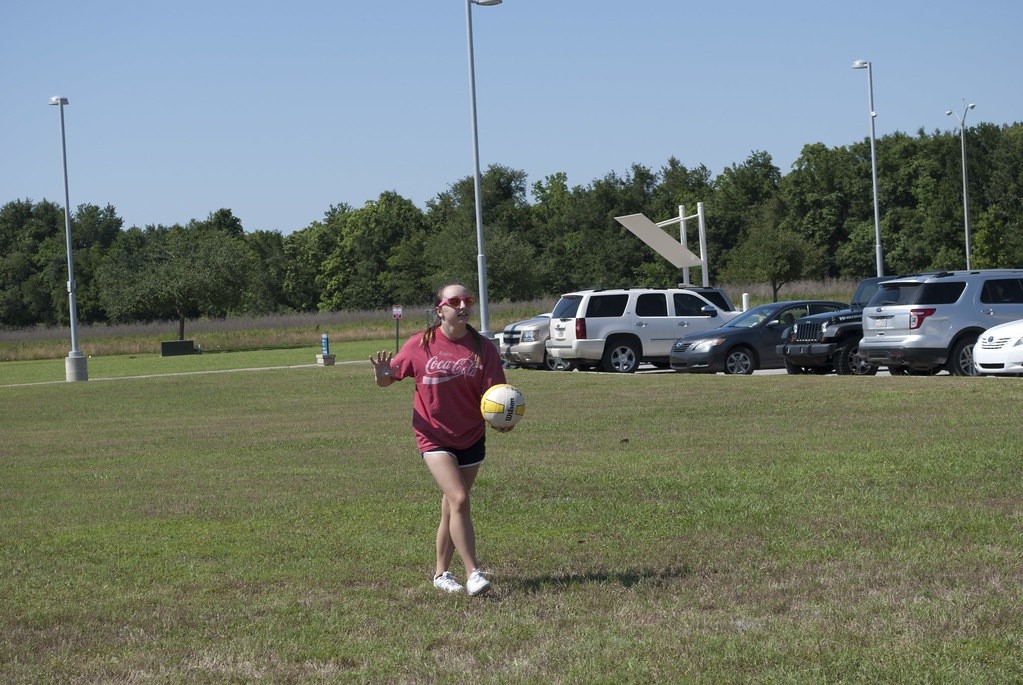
[369,282,514,595]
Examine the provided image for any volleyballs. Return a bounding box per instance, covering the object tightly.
[481,383,526,428]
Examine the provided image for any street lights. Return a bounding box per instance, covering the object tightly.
[850,59,888,276]
[48,95,89,383]
[466,0,510,359]
[945,103,977,271]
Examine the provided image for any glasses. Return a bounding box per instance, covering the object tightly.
[438,296,475,309]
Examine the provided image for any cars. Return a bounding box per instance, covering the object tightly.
[971,318,1023,378]
[501,313,576,371]
[670,300,849,374]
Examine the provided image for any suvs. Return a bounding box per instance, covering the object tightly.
[545,286,767,375]
[858,269,1022,377]
[782,274,920,378]
[675,287,736,312]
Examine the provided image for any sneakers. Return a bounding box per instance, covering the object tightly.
[466,569,491,597]
[434,571,464,593]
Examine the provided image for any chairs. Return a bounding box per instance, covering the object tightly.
[784,313,795,323]
[1001,283,1019,298]
